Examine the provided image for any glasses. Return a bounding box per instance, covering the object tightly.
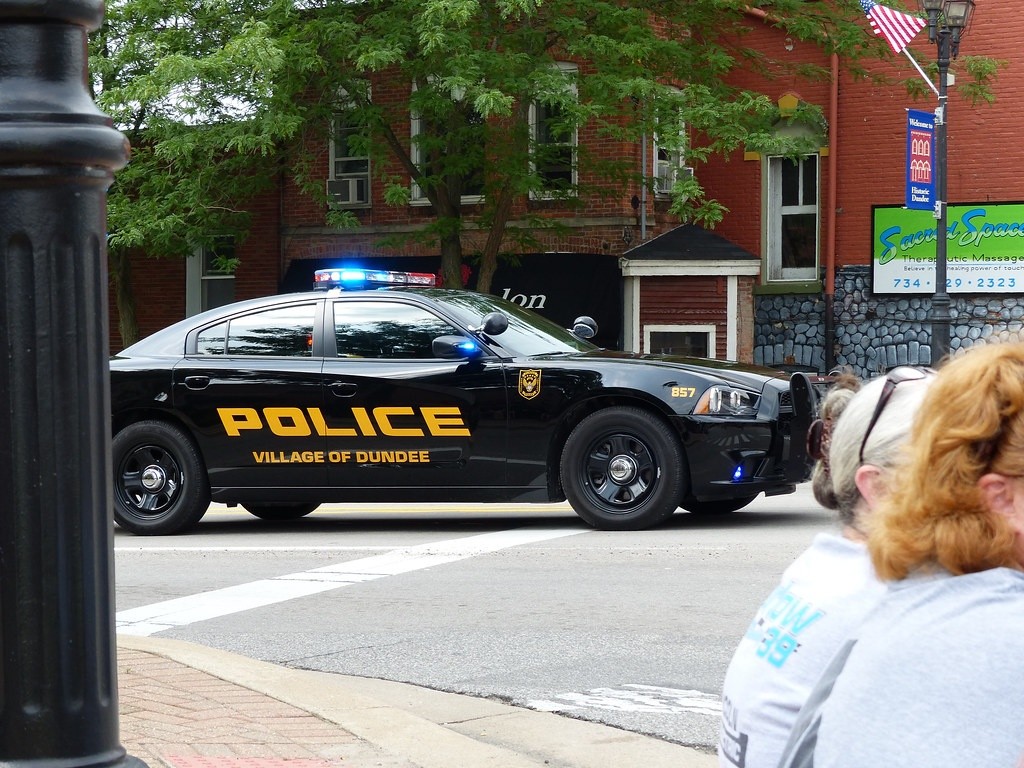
[859,362,937,462]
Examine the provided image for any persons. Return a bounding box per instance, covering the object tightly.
[779,344,1024,768]
[720,368,938,768]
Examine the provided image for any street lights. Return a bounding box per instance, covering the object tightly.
[915,1,978,368]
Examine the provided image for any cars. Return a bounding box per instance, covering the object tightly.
[107,267,802,532]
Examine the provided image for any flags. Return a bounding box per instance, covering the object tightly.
[861,0,926,58]
